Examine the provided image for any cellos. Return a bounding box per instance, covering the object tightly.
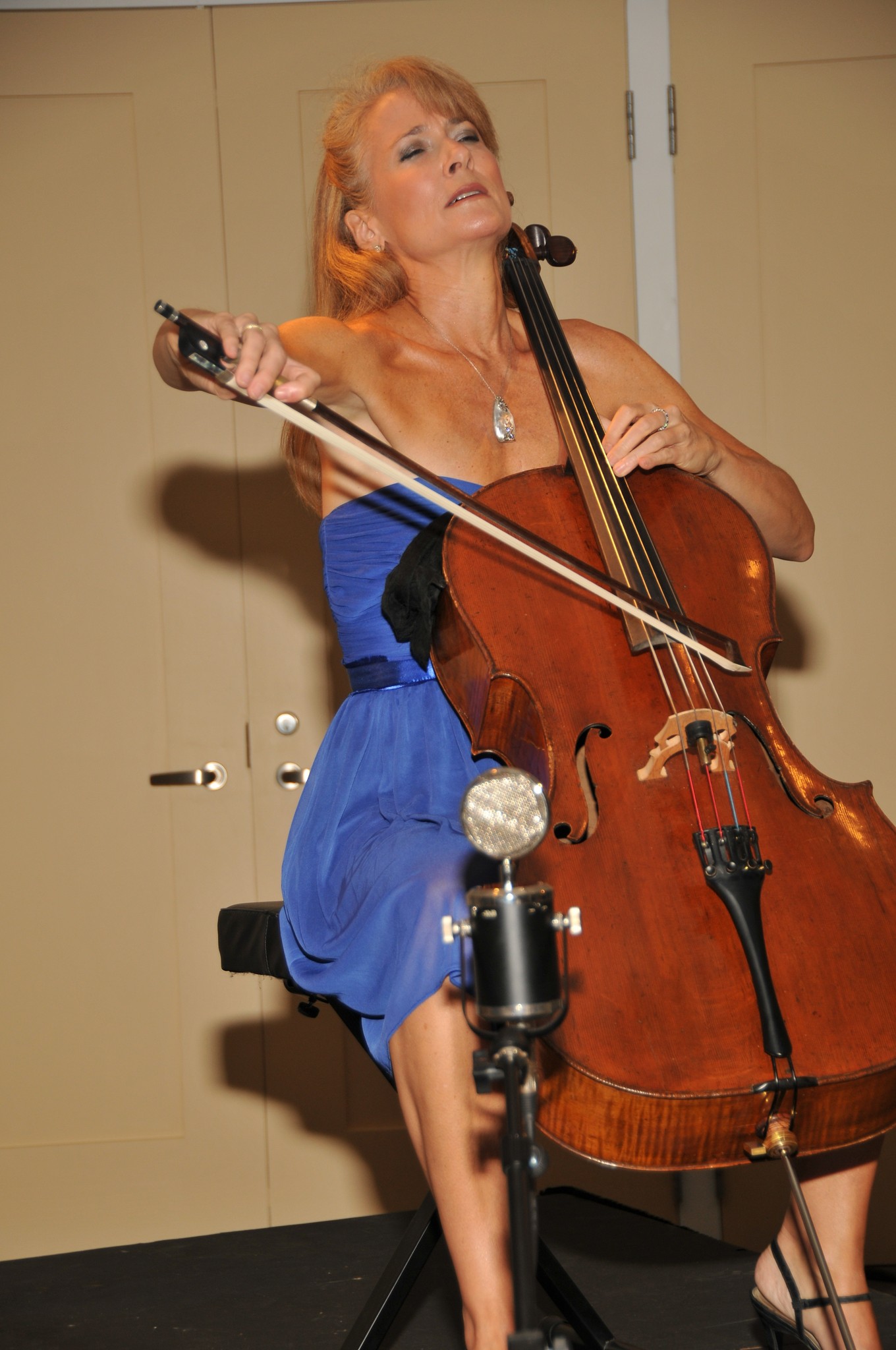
[381,187,894,1349]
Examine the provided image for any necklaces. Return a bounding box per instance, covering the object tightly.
[400,296,516,442]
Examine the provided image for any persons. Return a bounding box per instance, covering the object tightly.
[149,52,887,1350]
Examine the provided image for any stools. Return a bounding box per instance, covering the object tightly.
[219,903,642,1349]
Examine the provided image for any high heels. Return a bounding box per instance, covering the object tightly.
[748,1244,878,1350]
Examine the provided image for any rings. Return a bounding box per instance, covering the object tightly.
[242,325,263,335]
[652,408,670,430]
